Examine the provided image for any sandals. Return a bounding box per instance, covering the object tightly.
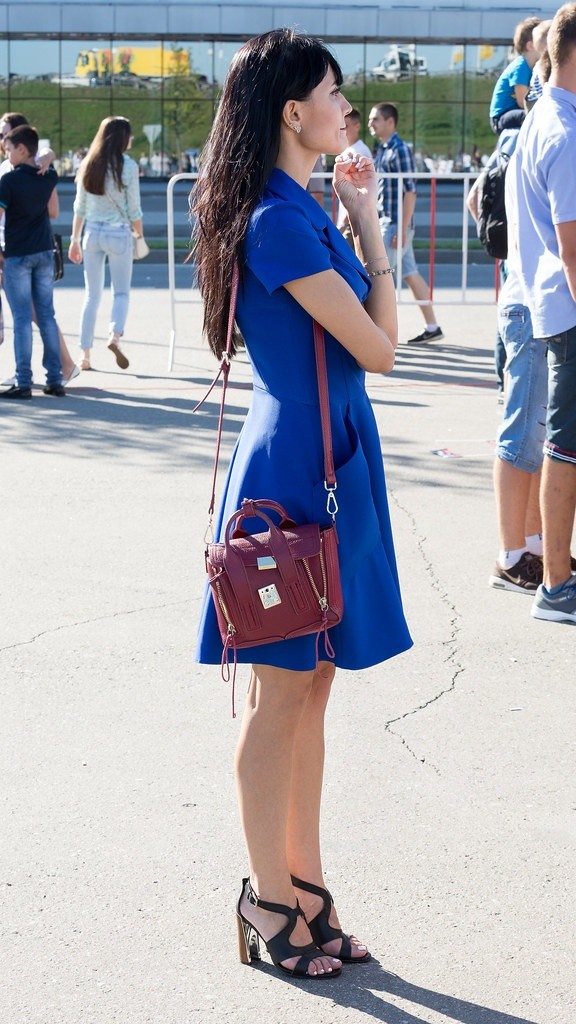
[108,337,129,369]
[80,358,91,370]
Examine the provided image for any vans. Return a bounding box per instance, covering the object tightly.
[373,49,429,82]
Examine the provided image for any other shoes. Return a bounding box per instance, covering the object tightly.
[1,375,33,386]
[44,384,64,396]
[60,365,80,385]
[0,385,32,399]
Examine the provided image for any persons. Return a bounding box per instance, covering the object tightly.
[139,149,199,177]
[69,116,145,371]
[336,110,371,246]
[0,113,80,398]
[368,102,445,344]
[309,154,326,207]
[71,145,89,175]
[196,31,413,980]
[467,3,575,624]
[472,151,481,167]
[489,18,542,132]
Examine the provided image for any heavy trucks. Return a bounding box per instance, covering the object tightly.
[74,48,190,78]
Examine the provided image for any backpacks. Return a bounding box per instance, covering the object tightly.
[476,161,510,257]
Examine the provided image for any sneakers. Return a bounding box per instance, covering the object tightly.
[490,550,542,594]
[532,576,576,622]
[408,327,442,345]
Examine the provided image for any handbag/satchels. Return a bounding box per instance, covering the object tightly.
[206,498,343,648]
[133,231,149,260]
[53,233,64,281]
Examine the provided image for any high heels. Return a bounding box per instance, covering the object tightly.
[292,877,371,963]
[236,877,340,977]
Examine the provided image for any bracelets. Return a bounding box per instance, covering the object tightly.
[70,236,81,243]
[364,257,388,266]
[368,268,395,277]
